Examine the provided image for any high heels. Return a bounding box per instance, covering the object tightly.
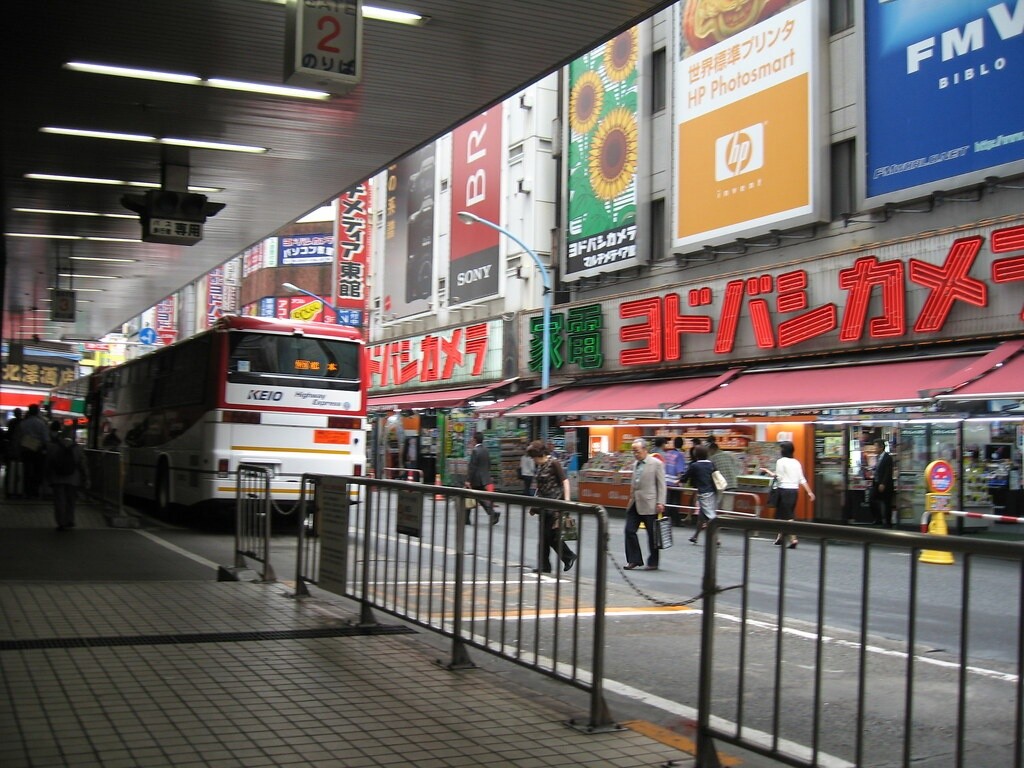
[786,537,798,549]
[774,534,782,544]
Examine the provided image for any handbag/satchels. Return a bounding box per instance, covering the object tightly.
[462,486,476,509]
[20,435,42,452]
[653,511,673,549]
[767,476,780,508]
[555,516,578,541]
[711,462,727,494]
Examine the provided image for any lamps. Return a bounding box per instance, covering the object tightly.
[599,271,641,279]
[375,312,392,330]
[542,286,582,295]
[38,123,274,155]
[62,59,333,104]
[578,275,618,285]
[381,314,401,327]
[424,407,439,416]
[399,408,416,419]
[23,173,226,194]
[839,212,893,229]
[391,313,423,322]
[736,238,781,248]
[255,0,432,28]
[932,186,984,203]
[449,296,488,309]
[703,245,748,255]
[438,300,454,310]
[984,175,1024,193]
[884,197,935,214]
[673,252,714,268]
[425,302,460,313]
[769,228,818,239]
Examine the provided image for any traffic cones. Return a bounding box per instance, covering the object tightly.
[405,471,415,482]
[434,474,446,500]
[486,482,495,505]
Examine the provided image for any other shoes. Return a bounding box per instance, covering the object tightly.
[623,562,643,569]
[464,521,470,525]
[532,568,552,573]
[643,565,658,570]
[564,555,577,571]
[492,513,500,524]
[56,526,64,531]
[688,537,697,543]
[65,522,74,527]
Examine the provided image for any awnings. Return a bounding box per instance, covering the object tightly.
[917,339,1024,403]
[366,381,510,408]
[476,354,985,416]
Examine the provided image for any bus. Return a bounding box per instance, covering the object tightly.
[49,312,373,528]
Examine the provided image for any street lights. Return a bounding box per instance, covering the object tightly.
[456,210,552,443]
[281,282,348,324]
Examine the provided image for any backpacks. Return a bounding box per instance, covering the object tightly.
[53,440,77,475]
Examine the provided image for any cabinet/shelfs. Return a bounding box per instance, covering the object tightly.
[850,439,1024,532]
[574,427,782,517]
[416,409,579,492]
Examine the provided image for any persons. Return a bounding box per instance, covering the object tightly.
[623,438,666,571]
[673,446,721,551]
[464,432,500,526]
[520,453,536,496]
[524,441,577,572]
[650,435,742,527]
[864,438,893,530]
[758,440,814,549]
[0,404,92,532]
